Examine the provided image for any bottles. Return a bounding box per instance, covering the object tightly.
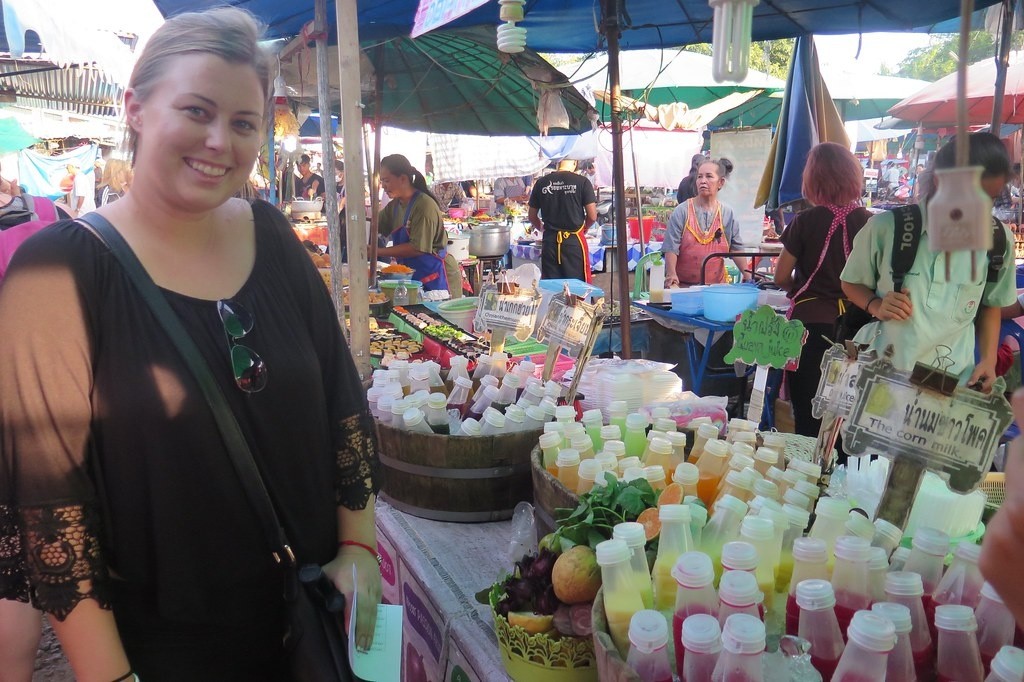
[539,400,1024,682]
[368,353,563,436]
[393,280,409,307]
[649,255,664,303]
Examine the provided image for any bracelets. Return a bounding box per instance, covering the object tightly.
[111,668,133,682]
[132,673,140,682]
[866,295,882,318]
[337,540,382,566]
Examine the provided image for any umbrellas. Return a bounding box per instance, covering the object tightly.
[152,0,1024,358]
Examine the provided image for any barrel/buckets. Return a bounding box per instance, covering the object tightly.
[370,419,542,521]
[437,296,479,334]
[626,216,657,244]
[601,222,618,245]
[448,208,465,219]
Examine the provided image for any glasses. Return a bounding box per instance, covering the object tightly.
[218,297,268,396]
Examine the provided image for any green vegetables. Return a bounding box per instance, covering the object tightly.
[548,471,657,553]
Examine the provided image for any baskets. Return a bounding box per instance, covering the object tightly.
[488,573,597,682]
[483,334,576,382]
[389,313,476,371]
[558,399,583,422]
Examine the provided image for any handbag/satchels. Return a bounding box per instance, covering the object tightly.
[275,554,362,682]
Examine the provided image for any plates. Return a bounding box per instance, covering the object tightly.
[514,239,542,249]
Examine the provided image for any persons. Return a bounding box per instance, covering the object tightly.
[493,176,528,214]
[527,159,597,284]
[661,159,752,411]
[0,7,390,682]
[428,179,467,214]
[774,132,1024,633]
[676,155,705,204]
[581,162,604,197]
[522,176,533,196]
[366,154,463,299]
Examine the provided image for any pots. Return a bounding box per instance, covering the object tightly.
[468,220,513,257]
[291,197,324,212]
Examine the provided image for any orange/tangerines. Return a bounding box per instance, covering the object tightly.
[636,484,684,543]
[505,612,554,633]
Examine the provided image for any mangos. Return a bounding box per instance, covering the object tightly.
[552,544,601,603]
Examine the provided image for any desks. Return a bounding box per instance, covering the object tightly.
[631,282,794,433]
[510,239,661,273]
[591,298,650,360]
[477,256,504,283]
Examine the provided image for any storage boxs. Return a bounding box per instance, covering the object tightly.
[539,278,604,305]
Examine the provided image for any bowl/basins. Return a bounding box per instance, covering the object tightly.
[343,268,422,317]
[671,283,788,315]
[702,286,760,322]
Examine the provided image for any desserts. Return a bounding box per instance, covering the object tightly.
[345,306,491,360]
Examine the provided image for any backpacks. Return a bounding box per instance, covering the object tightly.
[0,194,60,283]
[833,204,1006,349]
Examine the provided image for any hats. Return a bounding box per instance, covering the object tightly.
[63,158,81,169]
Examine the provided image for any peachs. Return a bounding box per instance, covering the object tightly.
[312,253,331,267]
